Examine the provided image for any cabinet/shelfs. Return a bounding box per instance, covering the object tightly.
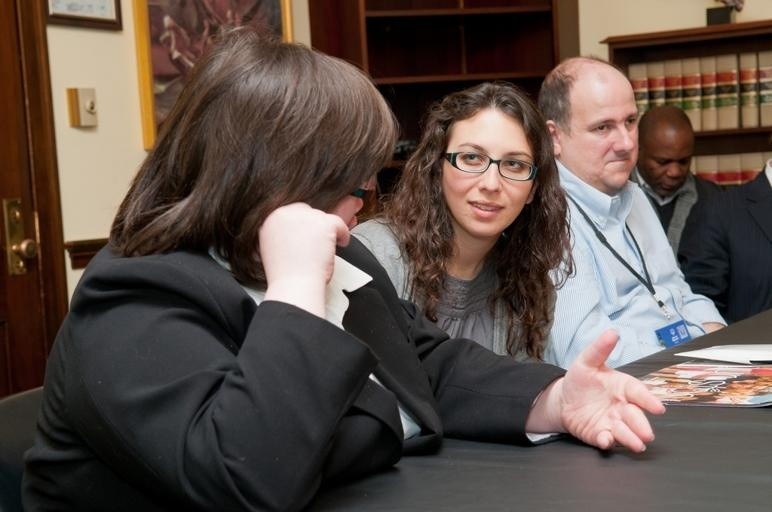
[344,1,578,171]
[600,19,772,185]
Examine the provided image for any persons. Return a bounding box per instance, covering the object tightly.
[348,80,578,364]
[20,27,667,512]
[623,106,723,256]
[676,160,772,322]
[537,55,727,369]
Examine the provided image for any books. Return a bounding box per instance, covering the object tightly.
[628,50,772,186]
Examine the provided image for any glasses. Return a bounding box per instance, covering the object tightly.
[441,151,540,182]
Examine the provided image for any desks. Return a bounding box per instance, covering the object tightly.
[313,309,772,512]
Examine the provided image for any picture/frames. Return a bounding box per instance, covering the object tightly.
[45,0,122,30]
[132,0,294,151]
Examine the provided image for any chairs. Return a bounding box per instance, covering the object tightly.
[0,387,43,512]
